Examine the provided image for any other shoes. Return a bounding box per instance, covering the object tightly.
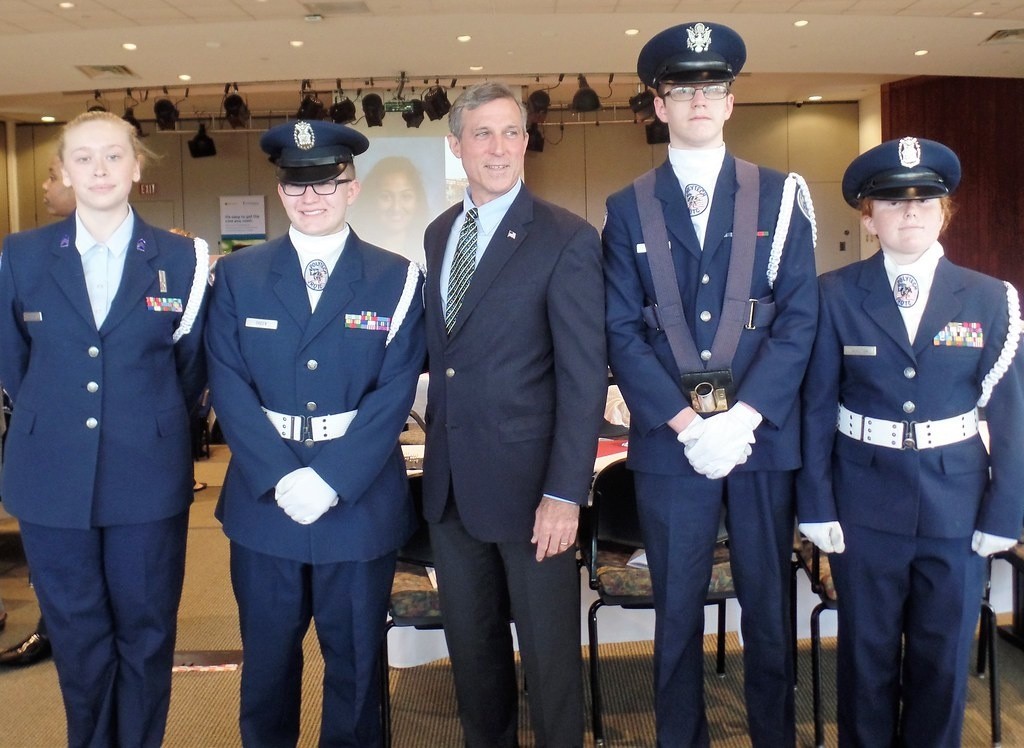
[193,483,207,491]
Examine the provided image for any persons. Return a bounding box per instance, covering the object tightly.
[0,111,211,748]
[203,119,425,748]
[600,21,822,748]
[419,82,607,748]
[798,138,1024,748]
[351,157,426,263]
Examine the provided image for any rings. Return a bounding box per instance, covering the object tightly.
[561,543,568,545]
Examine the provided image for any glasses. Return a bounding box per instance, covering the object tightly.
[660,84,730,101]
[278,179,352,196]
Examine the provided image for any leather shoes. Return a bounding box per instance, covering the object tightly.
[0,633,49,666]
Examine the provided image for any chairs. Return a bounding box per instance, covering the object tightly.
[372,458,1024,748]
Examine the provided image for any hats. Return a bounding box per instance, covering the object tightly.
[637,22,747,91]
[259,120,369,185]
[842,137,961,210]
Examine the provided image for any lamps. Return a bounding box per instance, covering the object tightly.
[76,69,673,160]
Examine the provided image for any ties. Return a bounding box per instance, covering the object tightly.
[445,208,479,340]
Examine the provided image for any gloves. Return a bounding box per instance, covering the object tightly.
[677,403,762,480]
[971,529,1019,557]
[274,467,339,525]
[798,521,845,554]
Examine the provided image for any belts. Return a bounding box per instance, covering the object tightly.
[260,406,358,443]
[836,402,979,452]
